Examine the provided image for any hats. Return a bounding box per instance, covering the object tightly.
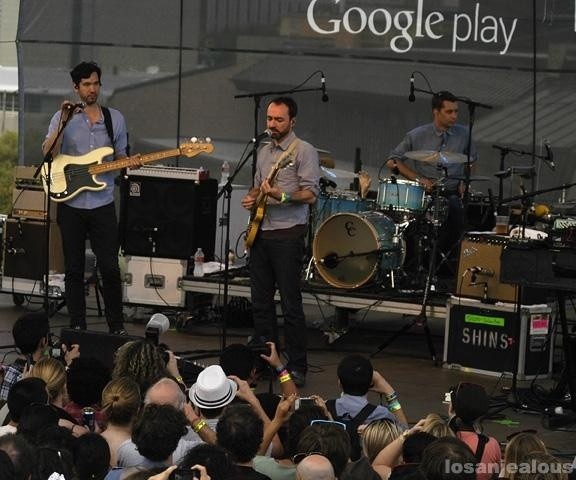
[188,364,238,409]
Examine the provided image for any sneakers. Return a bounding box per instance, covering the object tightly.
[114,328,128,336]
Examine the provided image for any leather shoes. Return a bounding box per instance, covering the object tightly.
[288,369,305,386]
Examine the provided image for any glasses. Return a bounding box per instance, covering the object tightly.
[455,381,485,398]
[310,420,346,431]
[292,452,322,464]
[507,429,537,441]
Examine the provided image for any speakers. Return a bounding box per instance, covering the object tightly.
[60,327,146,378]
[2,219,67,280]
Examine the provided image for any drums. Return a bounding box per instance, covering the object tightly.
[377,177,428,214]
[312,211,407,290]
[322,189,367,216]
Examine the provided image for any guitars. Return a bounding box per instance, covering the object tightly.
[41,138,215,202]
[245,150,294,247]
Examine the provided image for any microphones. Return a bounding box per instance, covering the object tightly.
[321,75,328,102]
[252,129,272,143]
[65,101,87,109]
[409,74,415,101]
[317,256,336,264]
[544,136,555,168]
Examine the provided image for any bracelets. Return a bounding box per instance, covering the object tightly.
[281,190,290,204]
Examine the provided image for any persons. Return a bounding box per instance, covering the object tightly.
[38,60,144,336]
[242,95,322,386]
[385,90,480,292]
[0,310,576,480]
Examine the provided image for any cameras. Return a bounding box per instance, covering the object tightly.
[49,331,76,360]
[294,398,317,411]
[441,392,452,404]
[249,336,271,360]
[169,468,200,480]
[144,312,169,368]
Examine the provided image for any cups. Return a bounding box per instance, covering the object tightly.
[495,215,509,234]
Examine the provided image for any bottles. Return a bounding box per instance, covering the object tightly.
[219,161,230,186]
[192,248,204,278]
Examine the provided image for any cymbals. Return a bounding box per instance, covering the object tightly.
[450,174,490,181]
[322,164,356,180]
[405,151,473,165]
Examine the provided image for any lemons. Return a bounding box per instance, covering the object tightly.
[535,203,551,216]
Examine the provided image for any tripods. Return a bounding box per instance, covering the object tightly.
[171,143,268,368]
[49,255,105,319]
[404,170,457,294]
[488,182,576,416]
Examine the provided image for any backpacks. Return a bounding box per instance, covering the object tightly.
[326,399,377,462]
